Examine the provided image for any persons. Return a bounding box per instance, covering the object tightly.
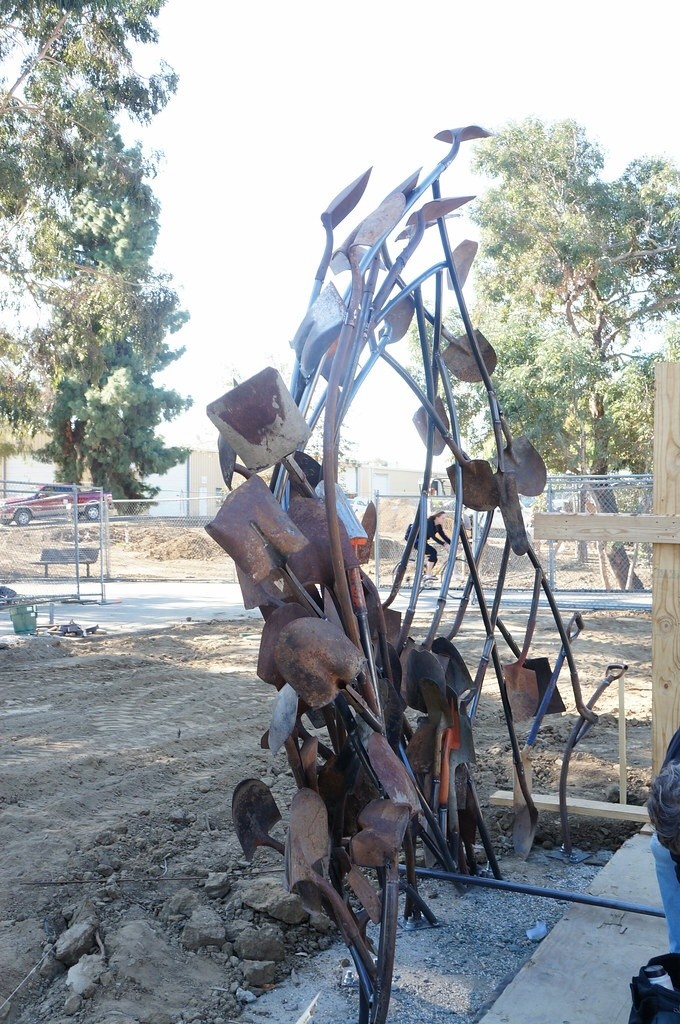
[646,725,680,953]
[414,510,451,580]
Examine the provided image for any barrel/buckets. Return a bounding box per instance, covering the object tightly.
[9,605,37,634]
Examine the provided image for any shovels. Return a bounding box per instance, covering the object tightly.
[203,119,629,1000]
[514,611,586,813]
[285,787,377,983]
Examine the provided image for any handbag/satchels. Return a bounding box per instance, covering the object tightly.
[625,953,680,1024]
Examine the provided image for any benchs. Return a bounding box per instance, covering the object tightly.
[29,548,101,578]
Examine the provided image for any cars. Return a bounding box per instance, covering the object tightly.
[1,483,113,527]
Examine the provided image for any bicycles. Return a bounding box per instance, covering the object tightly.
[391,547,469,599]
